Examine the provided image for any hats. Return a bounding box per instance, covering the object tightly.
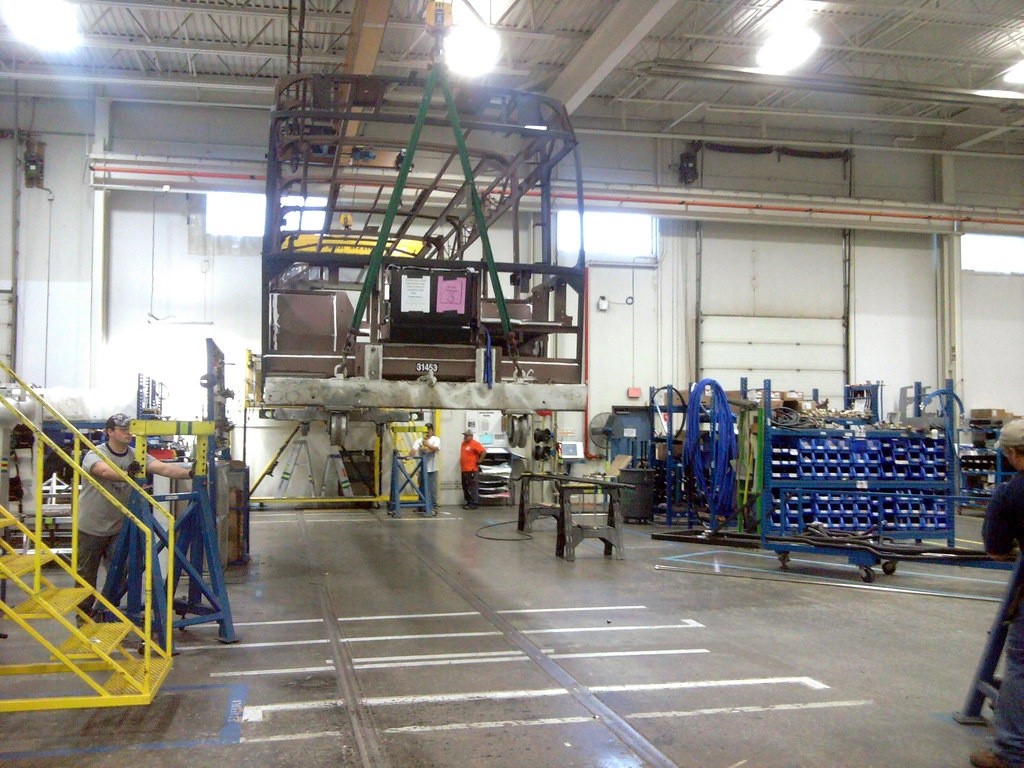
[106,414,135,430]
[425,423,434,431]
[993,420,1024,449]
[462,430,474,437]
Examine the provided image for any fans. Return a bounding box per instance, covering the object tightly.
[589,412,625,462]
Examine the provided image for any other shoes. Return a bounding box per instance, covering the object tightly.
[77,617,87,628]
[461,503,476,510]
[970,749,1024,768]
[431,508,438,516]
[413,507,425,514]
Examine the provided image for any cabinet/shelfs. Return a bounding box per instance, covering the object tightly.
[650,379,1016,547]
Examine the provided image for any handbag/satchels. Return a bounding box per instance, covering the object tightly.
[9,476,23,502]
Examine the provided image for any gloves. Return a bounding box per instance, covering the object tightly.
[128,461,141,478]
[189,461,208,478]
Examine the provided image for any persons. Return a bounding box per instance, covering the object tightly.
[969,416,1024,767]
[409,423,440,516]
[460,428,486,509]
[76,413,207,624]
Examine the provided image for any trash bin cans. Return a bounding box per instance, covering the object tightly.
[619,468,656,524]
